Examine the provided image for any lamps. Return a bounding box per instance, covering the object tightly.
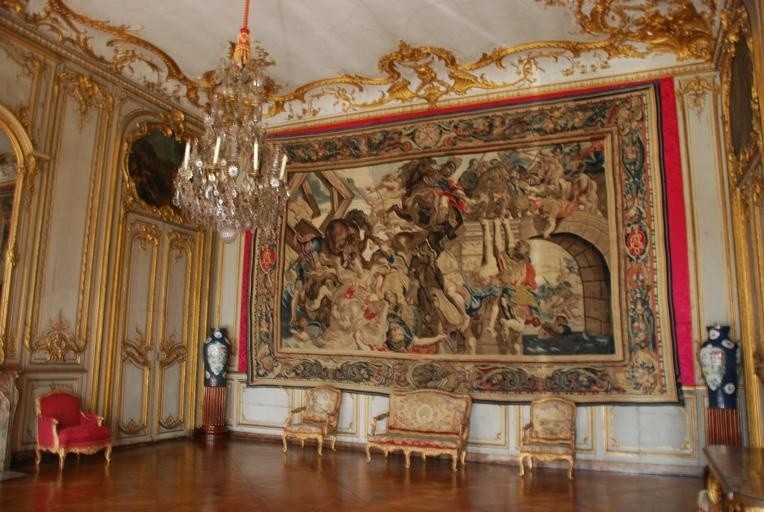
[172,1,291,244]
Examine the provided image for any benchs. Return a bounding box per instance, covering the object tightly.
[365,388,472,472]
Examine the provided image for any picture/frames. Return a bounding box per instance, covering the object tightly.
[120,110,194,226]
[720,7,762,190]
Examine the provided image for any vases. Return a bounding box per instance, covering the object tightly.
[203,327,232,386]
[696,324,739,410]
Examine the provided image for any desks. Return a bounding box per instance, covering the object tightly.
[703,443,764,512]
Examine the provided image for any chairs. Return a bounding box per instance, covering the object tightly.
[515,397,577,481]
[35,390,114,473]
[281,385,343,456]
